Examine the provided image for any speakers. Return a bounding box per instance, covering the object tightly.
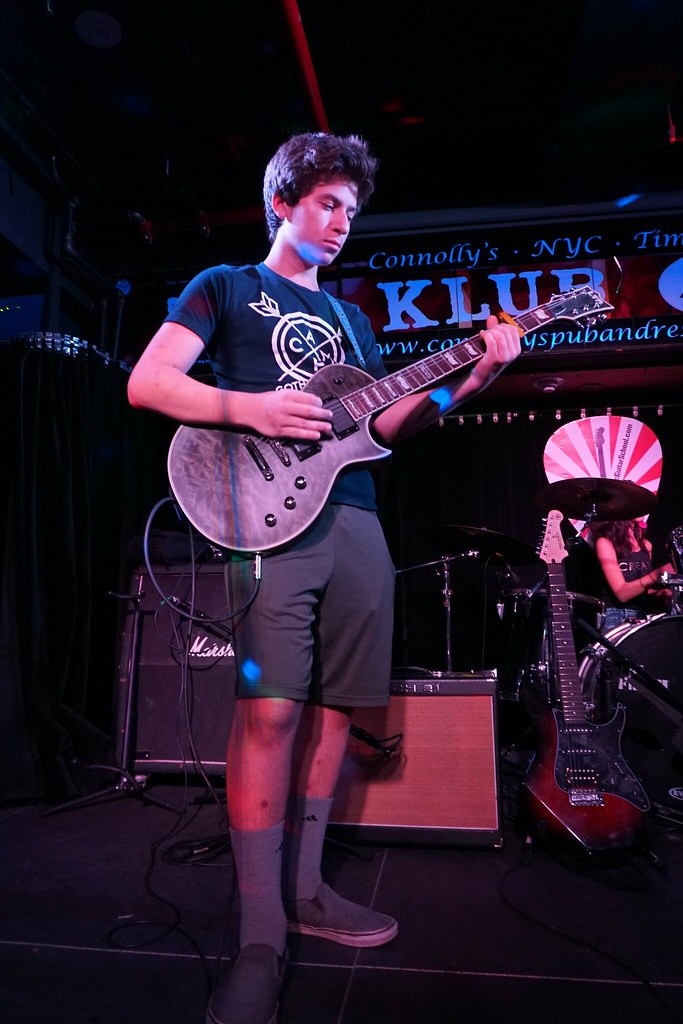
[114,563,236,779]
[327,674,502,853]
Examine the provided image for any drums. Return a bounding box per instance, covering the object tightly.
[486,583,606,664]
[580,615,683,819]
[665,522,683,576]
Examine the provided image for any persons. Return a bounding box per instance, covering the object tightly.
[591,516,678,639]
[127,133,522,1022]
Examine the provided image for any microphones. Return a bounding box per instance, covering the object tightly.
[166,595,192,608]
[381,743,402,759]
[495,553,521,586]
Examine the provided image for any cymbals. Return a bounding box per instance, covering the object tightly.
[420,524,539,568]
[534,477,658,522]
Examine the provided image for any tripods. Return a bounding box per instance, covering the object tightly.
[35,576,187,820]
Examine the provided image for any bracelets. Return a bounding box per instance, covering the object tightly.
[640,578,646,589]
[649,573,657,583]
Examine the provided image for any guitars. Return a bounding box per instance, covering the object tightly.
[519,510,650,873]
[167,284,615,552]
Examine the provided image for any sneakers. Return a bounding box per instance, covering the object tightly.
[205,943,288,1024]
[283,884,400,948]
